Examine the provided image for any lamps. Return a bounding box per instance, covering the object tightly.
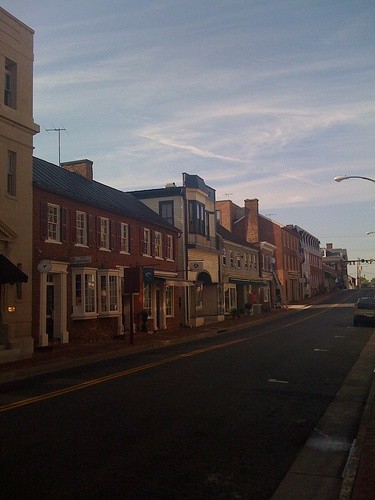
[7,302,16,314]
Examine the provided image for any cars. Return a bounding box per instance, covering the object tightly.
[354,296,375,327]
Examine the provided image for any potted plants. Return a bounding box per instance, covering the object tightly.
[139,309,152,332]
[229,301,253,321]
